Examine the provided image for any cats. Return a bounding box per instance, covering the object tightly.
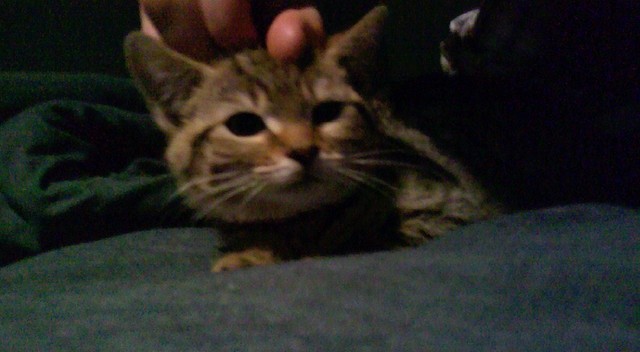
[123,5,520,272]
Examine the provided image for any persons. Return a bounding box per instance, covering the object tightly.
[139,0,325,67]
